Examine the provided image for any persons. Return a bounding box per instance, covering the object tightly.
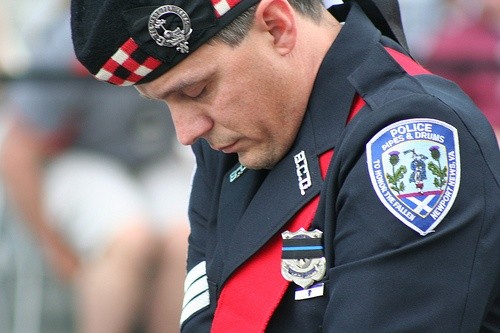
[0,10,197,333]
[70,0,500,333]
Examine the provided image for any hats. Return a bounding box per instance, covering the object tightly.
[71,0,262,86]
